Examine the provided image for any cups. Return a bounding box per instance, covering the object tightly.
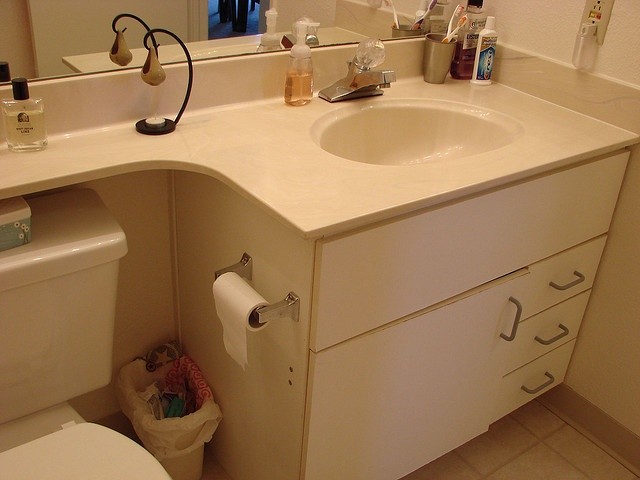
[391,25,424,38]
[423,33,458,84]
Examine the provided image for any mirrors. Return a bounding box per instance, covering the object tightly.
[0,0,459,85]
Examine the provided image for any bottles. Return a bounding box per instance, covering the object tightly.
[426,0,452,33]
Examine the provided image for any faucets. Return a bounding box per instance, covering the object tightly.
[320,36,396,103]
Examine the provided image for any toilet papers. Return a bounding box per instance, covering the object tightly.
[211,269,268,370]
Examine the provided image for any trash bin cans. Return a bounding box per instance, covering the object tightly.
[117,341,222,480]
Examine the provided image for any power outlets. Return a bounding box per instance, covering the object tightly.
[579,0,614,46]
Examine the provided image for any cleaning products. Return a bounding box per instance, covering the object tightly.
[5,72,46,151]
[255,7,282,52]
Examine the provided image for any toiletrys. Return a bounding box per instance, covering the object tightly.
[286,22,319,110]
[454,1,488,80]
[474,16,499,83]
[0,76,46,154]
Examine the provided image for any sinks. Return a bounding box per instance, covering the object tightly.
[317,95,507,170]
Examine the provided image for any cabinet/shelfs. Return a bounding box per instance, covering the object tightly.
[297,151,639,478]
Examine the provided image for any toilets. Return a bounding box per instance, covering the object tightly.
[0,192,173,480]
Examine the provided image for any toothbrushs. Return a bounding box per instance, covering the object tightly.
[410,0,438,30]
[449,17,470,43]
[384,0,400,30]
[442,1,465,40]
